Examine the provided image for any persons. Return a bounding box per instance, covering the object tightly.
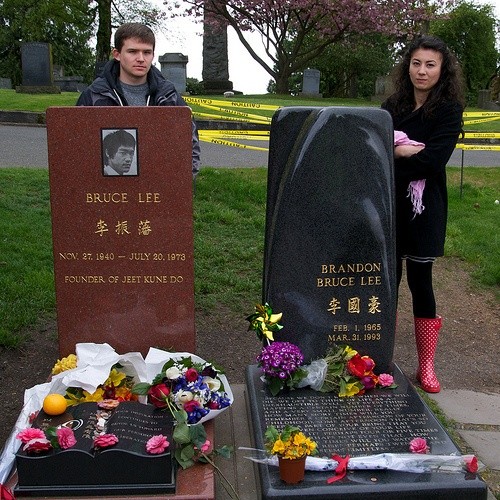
[381,36,464,393]
[75,23,199,184]
[102,130,135,175]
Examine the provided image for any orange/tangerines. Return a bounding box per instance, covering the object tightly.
[43,394,67,415]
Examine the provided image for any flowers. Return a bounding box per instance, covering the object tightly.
[172,410,241,500]
[257,343,397,397]
[47,353,133,405]
[248,444,485,477]
[409,434,429,454]
[130,360,233,422]
[266,421,319,484]
[17,426,171,454]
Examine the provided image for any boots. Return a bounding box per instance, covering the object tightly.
[414,313,441,392]
